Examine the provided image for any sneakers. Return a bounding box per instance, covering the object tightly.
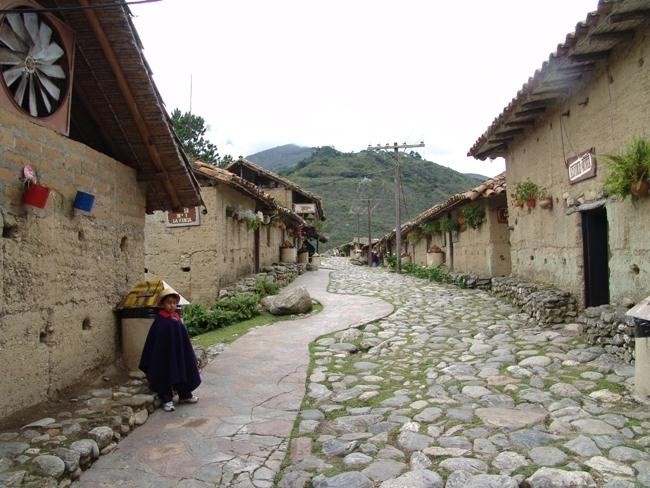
[178,395,200,403]
[162,400,176,412]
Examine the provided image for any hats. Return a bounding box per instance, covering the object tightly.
[156,288,180,306]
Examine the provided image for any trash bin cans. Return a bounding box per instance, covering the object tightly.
[426,245,445,268]
[401,253,411,263]
[624,296,650,405]
[113,281,192,372]
[280,240,297,263]
[298,248,309,263]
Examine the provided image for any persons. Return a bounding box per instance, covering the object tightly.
[136,287,203,413]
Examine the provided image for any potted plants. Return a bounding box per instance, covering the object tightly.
[406,204,486,246]
[514,177,542,207]
[592,135,650,209]
[21,165,66,212]
